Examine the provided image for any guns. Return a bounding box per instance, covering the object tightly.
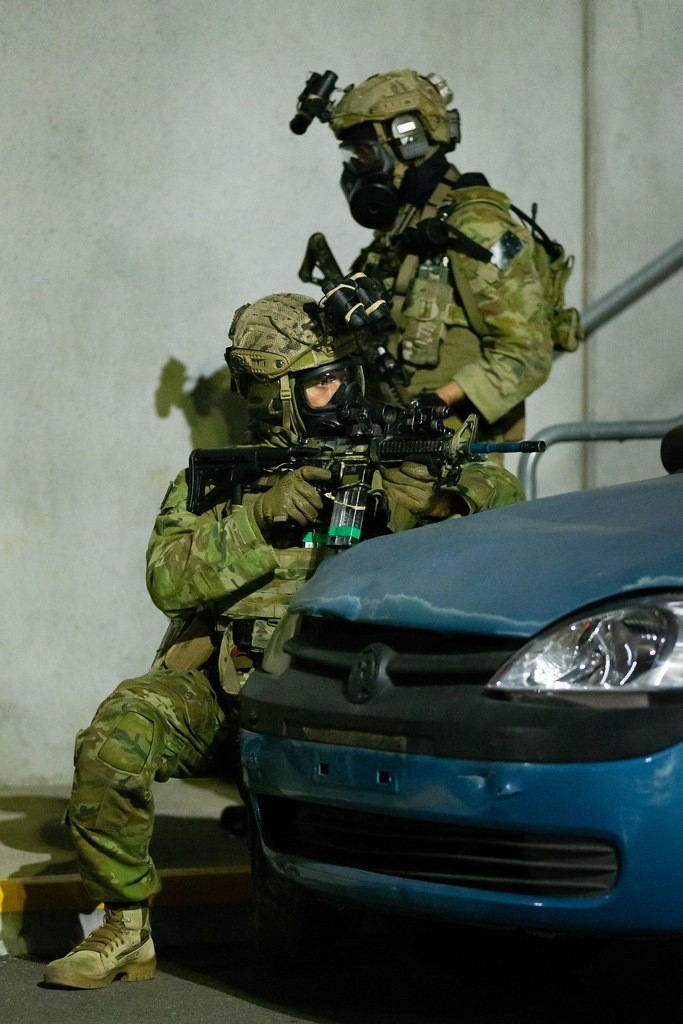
[297,231,447,479]
[182,404,549,550]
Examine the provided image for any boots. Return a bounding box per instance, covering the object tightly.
[43,902,158,988]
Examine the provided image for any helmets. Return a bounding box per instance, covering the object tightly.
[330,70,461,144]
[225,292,355,379]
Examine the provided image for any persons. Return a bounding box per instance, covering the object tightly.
[327,68,554,469]
[44,293,527,989]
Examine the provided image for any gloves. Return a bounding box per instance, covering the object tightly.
[380,461,449,517]
[254,466,332,528]
[416,389,446,408]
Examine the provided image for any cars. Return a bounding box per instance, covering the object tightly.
[233,422,682,938]
